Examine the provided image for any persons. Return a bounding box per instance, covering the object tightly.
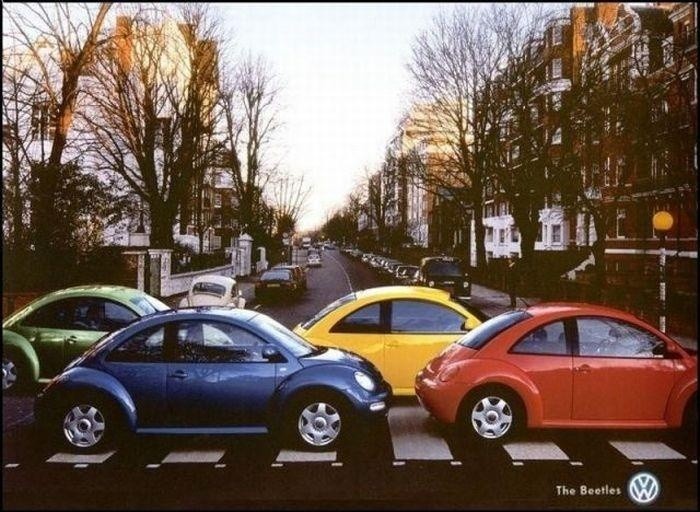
[280,251,286,266]
[178,251,191,273]
[508,258,518,310]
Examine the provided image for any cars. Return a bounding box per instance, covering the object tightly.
[254,236,472,305]
[291,285,492,397]
[1,282,237,396]
[415,301,699,448]
[177,274,247,312]
[33,305,393,456]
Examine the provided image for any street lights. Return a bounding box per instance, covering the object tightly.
[652,211,674,335]
